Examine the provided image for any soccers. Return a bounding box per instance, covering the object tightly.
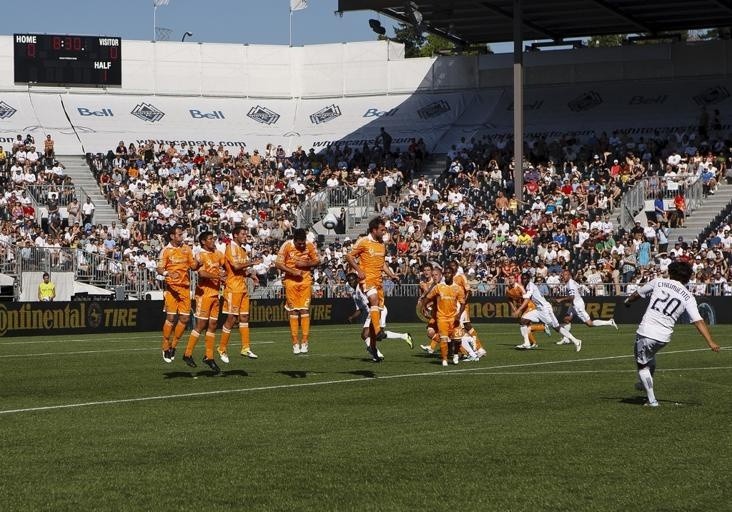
[322,212,338,229]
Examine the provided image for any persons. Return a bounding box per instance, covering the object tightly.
[556,271,620,344]
[156,224,263,374]
[506,272,582,352]
[275,227,320,355]
[419,261,488,366]
[0,127,730,308]
[349,217,414,362]
[625,262,720,407]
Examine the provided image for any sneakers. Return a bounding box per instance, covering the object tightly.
[557,337,570,344]
[367,331,415,362]
[301,343,308,353]
[530,343,538,348]
[576,339,582,352]
[516,344,530,349]
[420,345,487,366]
[609,318,618,330]
[293,344,300,354]
[644,399,658,406]
[163,347,258,371]
